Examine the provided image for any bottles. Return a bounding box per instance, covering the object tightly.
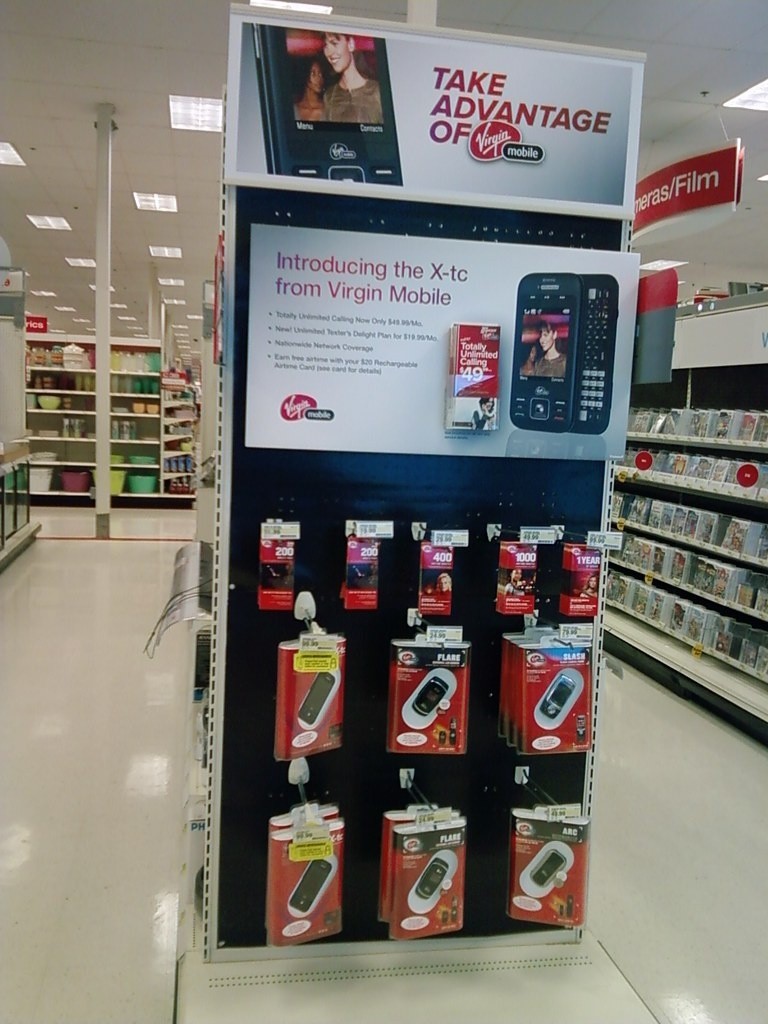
[61,414,86,438]
[111,350,160,372]
[29,345,62,368]
[111,418,136,440]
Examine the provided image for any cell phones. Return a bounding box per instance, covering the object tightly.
[289,858,332,913]
[510,272,583,433]
[415,858,449,899]
[530,850,567,887]
[568,273,618,435]
[411,676,449,717]
[297,671,335,725]
[253,22,403,187]
[540,675,576,720]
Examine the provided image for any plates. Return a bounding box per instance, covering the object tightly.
[39,430,59,436]
[110,455,124,463]
[129,455,156,464]
[32,452,57,460]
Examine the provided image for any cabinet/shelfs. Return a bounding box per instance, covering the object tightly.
[162,378,196,509]
[601,428,768,750]
[25,332,162,509]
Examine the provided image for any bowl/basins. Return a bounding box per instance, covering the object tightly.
[146,404,160,414]
[132,403,145,414]
[37,395,61,409]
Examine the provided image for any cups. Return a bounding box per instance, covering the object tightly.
[63,397,72,409]
[86,396,93,411]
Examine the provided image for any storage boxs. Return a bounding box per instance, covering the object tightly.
[606,407,768,684]
[444,323,501,431]
[257,533,604,950]
[183,625,209,952]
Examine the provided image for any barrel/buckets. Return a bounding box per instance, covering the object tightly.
[92,469,125,494]
[127,475,157,493]
[29,468,53,492]
[59,472,90,492]
